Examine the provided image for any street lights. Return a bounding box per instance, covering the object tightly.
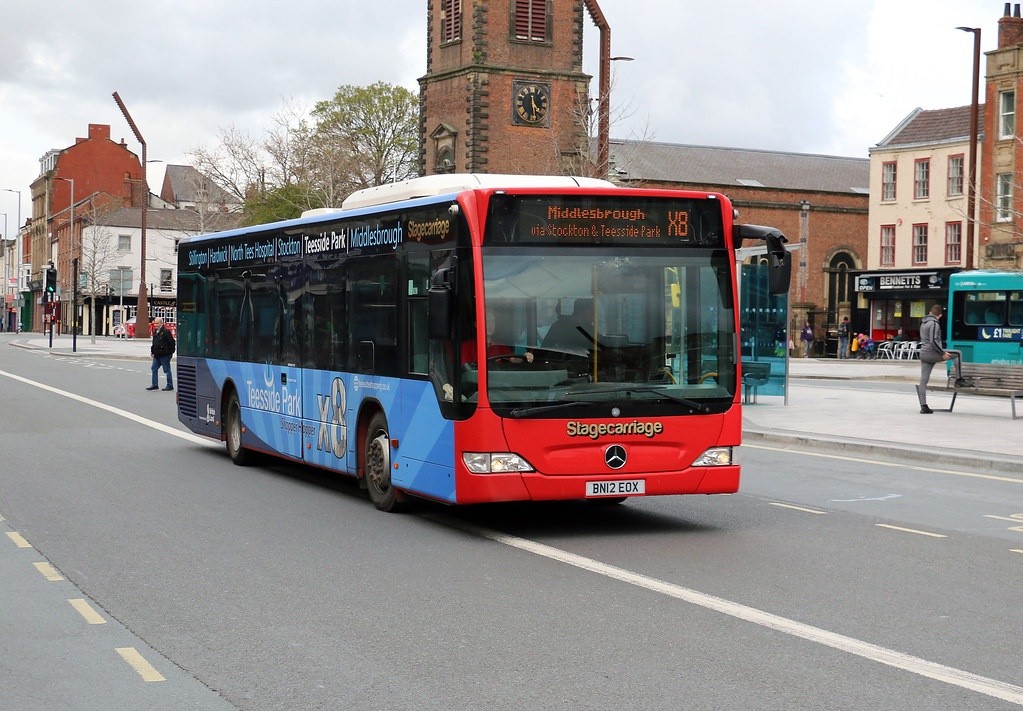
[51,177,73,332]
[955,27,982,271]
[2,188,21,333]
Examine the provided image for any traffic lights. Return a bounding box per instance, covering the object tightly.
[46,269,56,293]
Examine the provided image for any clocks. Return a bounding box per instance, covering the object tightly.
[514,84,549,123]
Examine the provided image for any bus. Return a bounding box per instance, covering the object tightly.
[176,172,791,513]
[947,270,1023,378]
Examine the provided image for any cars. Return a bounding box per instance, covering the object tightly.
[115,318,154,337]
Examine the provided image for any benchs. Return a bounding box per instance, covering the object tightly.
[701,360,770,404]
[943,361,1023,419]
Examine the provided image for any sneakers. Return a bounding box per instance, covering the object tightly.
[954,379,972,387]
[920,405,933,414]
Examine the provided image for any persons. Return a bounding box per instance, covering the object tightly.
[800,321,813,357]
[461,309,534,364]
[540,299,605,371]
[840,316,857,359]
[789,336,794,358]
[146,317,176,391]
[919,305,972,413]
[0,315,4,332]
[893,330,902,340]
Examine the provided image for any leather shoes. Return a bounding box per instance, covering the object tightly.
[161,384,173,391]
[146,385,158,390]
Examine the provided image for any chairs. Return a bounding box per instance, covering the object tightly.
[876,341,921,359]
[967,306,1000,324]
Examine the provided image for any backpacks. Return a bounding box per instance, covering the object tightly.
[837,322,848,337]
